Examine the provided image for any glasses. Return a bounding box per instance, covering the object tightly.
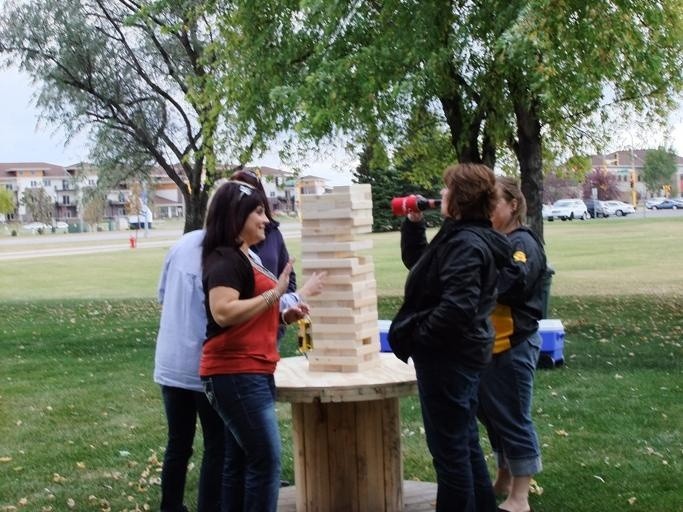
[236,185,252,202]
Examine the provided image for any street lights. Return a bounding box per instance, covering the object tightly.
[632,152,650,199]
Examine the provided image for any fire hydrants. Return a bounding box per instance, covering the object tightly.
[128,234,135,248]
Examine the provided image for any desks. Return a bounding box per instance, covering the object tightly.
[272,351,419,511]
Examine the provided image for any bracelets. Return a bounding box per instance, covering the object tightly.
[261,286,279,309]
[280,308,289,326]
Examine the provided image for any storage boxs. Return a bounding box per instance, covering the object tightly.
[536,319,566,368]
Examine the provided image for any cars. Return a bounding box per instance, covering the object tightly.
[544,194,681,222]
[21,220,68,235]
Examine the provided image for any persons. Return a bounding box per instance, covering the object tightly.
[231,172,297,349]
[194,179,313,511]
[475,176,547,512]
[386,163,511,511]
[152,227,329,512]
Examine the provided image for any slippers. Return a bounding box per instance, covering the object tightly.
[497,505,533,512]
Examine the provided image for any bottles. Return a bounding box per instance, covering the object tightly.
[390,194,443,216]
[296,295,313,352]
